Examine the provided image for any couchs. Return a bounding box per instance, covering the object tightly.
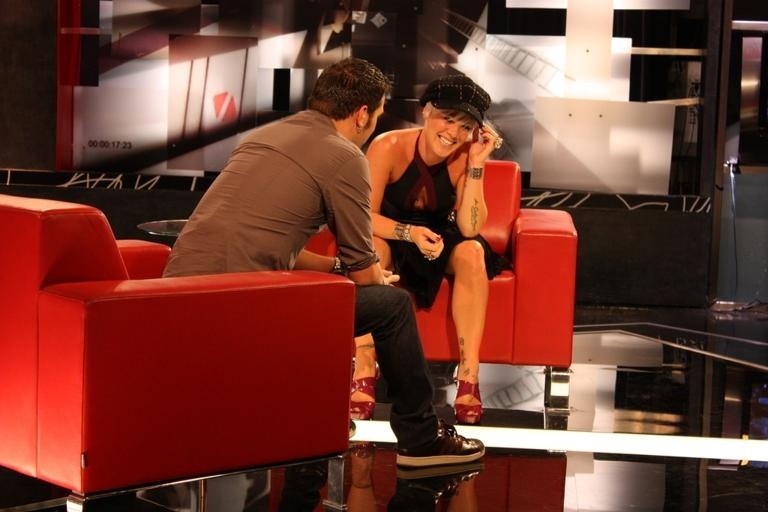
[274,159,579,417]
[2,187,357,512]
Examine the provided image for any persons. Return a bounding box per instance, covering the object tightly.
[349,440,478,512]
[349,74,501,424]
[163,55,484,468]
[277,460,482,512]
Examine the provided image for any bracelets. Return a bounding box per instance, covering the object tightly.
[394,221,413,244]
[465,167,483,179]
[329,256,341,273]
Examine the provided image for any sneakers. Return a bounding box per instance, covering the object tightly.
[349,419,357,438]
[287,461,328,493]
[396,461,485,504]
[395,419,486,468]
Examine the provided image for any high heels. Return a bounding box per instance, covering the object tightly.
[351,444,374,458]
[453,362,482,424]
[350,362,378,420]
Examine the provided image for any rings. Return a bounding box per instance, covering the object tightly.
[424,251,435,261]
[495,137,504,149]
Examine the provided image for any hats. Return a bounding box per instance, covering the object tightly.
[419,76,491,128]
